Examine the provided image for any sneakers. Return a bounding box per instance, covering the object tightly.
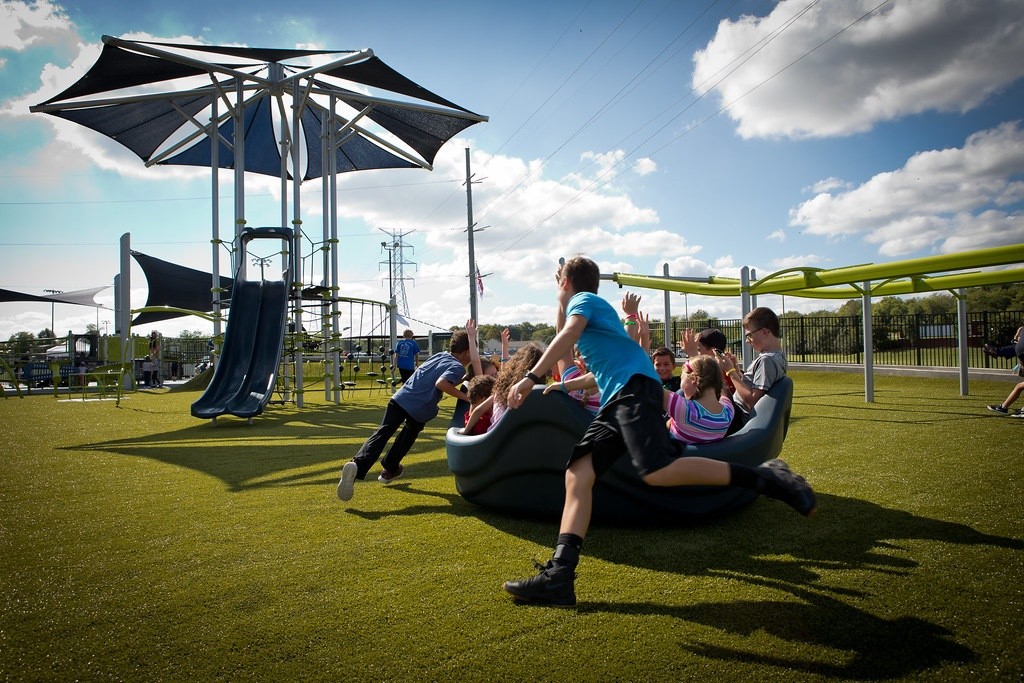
[336,462,358,501]
[377,464,404,482]
[1010,409,1024,418]
[503,558,577,608]
[757,457,819,516]
[987,404,1009,414]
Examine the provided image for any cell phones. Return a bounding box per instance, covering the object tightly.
[715,348,724,356]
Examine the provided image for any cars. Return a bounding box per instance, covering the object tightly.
[353,352,377,356]
[341,350,350,356]
[484,351,491,355]
[499,352,501,355]
[671,350,687,358]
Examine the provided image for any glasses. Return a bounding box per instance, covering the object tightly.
[746,327,769,340]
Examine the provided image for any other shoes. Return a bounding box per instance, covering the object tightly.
[984,343,999,350]
[983,348,998,359]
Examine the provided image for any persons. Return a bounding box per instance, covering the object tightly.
[455,291,789,445]
[337,328,479,500]
[149,332,159,357]
[392,331,420,384]
[983,327,1024,418]
[150,354,162,388]
[141,356,153,389]
[502,257,818,605]
[195,362,207,376]
[78,362,86,386]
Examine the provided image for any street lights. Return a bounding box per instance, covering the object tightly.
[680,292,690,328]
[44,289,63,341]
[380,241,399,371]
[102,320,111,336]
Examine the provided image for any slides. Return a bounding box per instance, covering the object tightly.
[190,266,295,427]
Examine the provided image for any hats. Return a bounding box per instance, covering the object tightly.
[698,329,726,349]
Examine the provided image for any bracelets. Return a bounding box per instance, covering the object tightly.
[559,380,567,394]
[502,358,509,362]
[726,368,736,376]
[525,370,543,387]
[624,321,635,327]
[625,313,637,320]
